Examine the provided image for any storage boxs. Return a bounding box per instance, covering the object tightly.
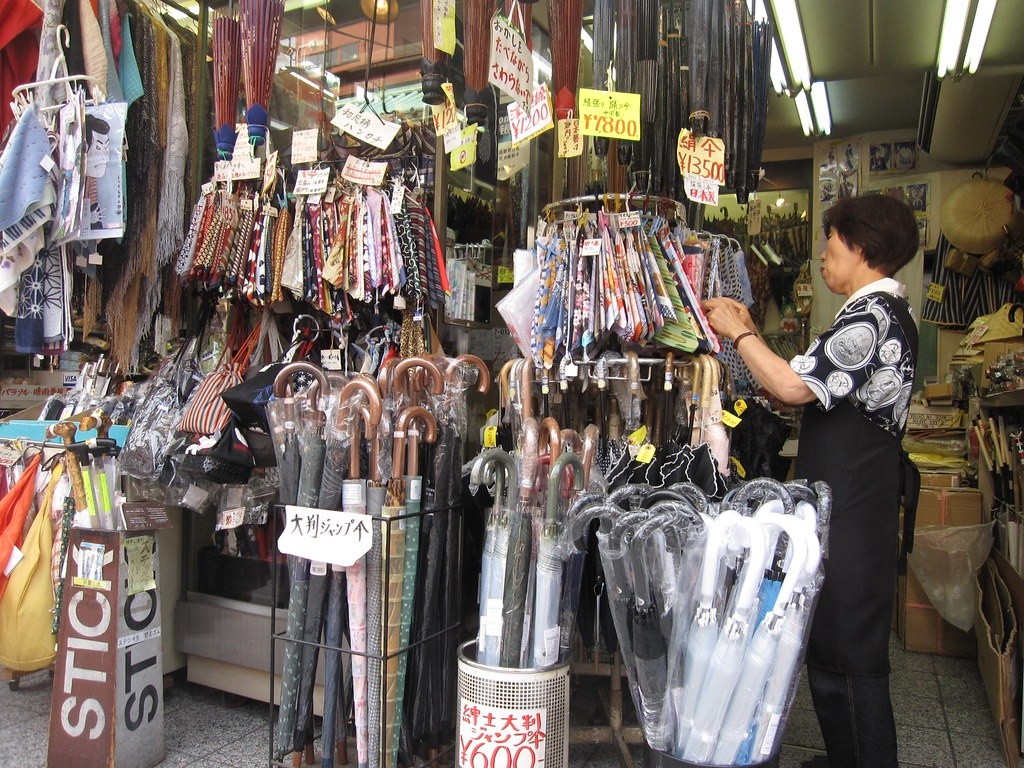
[895,469,984,653]
[0,399,131,449]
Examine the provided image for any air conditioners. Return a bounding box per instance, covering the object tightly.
[915,68,1024,165]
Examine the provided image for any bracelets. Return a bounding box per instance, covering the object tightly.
[732,330,758,355]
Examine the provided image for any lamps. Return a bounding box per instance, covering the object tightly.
[746,0,834,137]
[935,0,998,82]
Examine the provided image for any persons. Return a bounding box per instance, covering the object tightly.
[702,188,922,767]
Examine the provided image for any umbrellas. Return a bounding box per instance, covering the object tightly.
[211,0,286,160]
[264,353,832,768]
[418,1,774,229]
